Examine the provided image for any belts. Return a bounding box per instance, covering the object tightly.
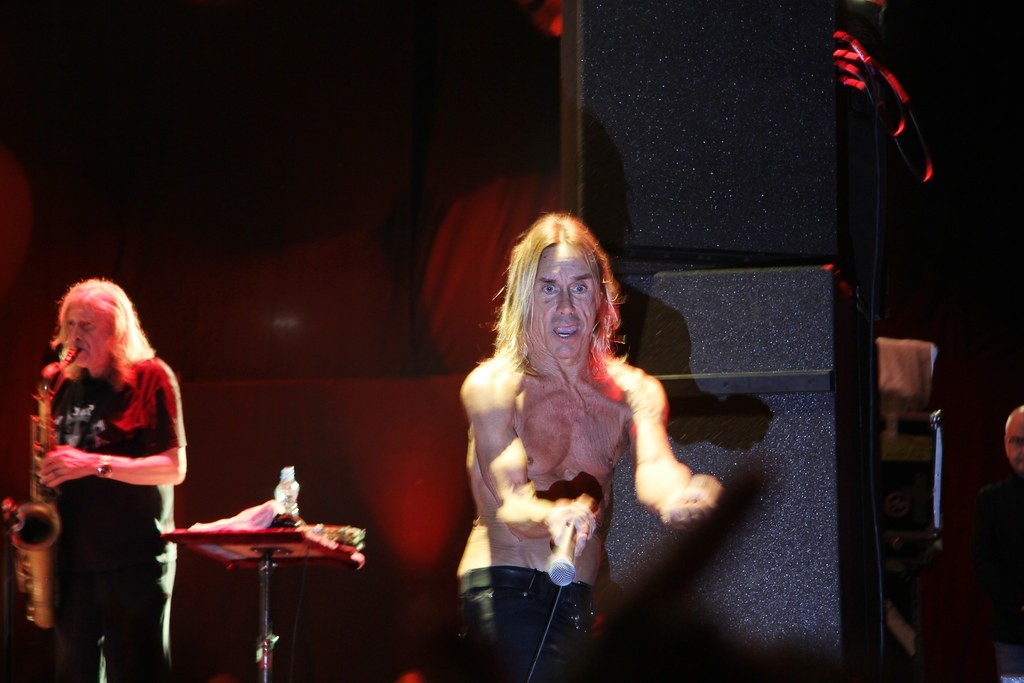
[459,565,597,616]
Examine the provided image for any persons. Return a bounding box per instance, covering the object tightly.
[457,210,725,683]
[969,404,1024,682]
[35,274,189,683]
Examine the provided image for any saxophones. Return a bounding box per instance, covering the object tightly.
[2,346,84,627]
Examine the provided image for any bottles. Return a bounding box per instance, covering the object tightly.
[274,466,299,519]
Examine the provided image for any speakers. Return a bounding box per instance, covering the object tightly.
[559,0,843,266]
[598,262,847,674]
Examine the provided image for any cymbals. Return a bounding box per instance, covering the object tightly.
[300,523,365,551]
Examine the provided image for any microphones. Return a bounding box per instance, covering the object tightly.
[549,494,594,586]
